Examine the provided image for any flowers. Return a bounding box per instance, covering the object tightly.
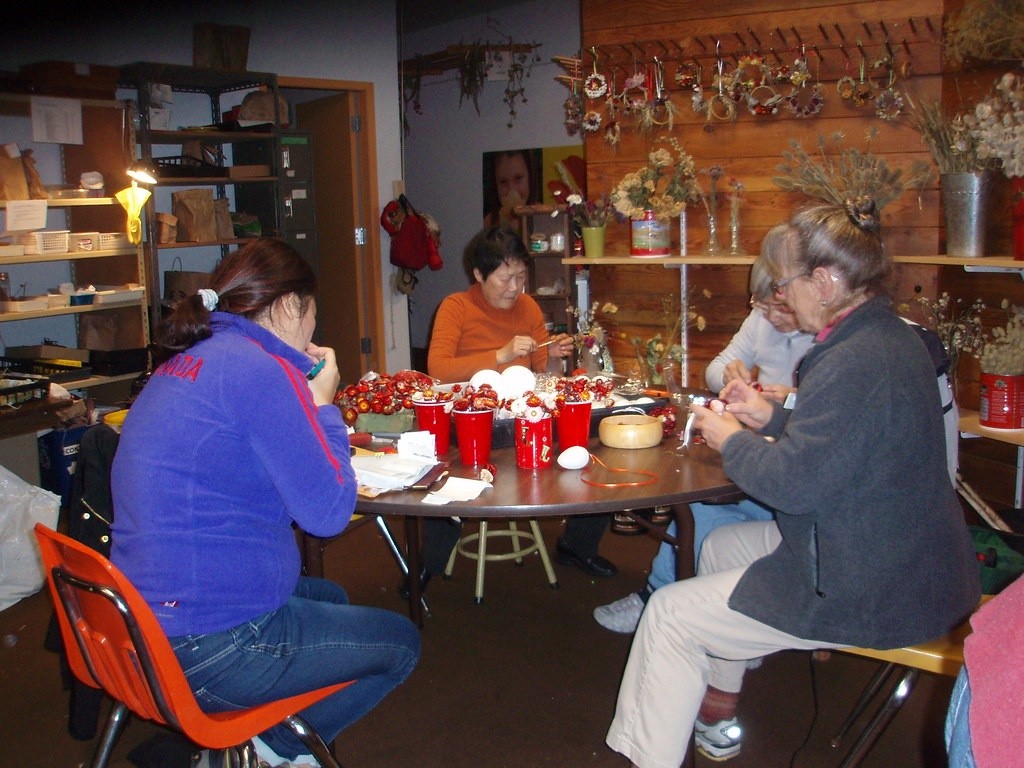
[963,72,1024,179]
[607,147,699,220]
[563,302,619,347]
[974,296,1024,379]
[697,165,722,216]
[722,178,747,218]
[898,291,986,368]
[548,191,614,227]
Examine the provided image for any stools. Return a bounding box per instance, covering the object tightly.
[828,594,996,768]
[442,515,560,604]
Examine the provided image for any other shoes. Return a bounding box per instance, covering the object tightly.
[193,739,260,768]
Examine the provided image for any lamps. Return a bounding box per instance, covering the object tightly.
[113,151,157,245]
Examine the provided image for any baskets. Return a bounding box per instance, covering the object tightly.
[0,375,42,409]
[154,156,223,176]
[0,358,79,372]
[12,229,135,255]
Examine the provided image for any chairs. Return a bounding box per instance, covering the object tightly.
[85,424,434,620]
[33,523,358,768]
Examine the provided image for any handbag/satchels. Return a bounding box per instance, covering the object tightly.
[165,255,223,301]
[393,194,431,270]
[37,419,100,501]
[155,186,234,247]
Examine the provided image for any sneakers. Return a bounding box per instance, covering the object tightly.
[693,714,741,763]
[593,585,653,635]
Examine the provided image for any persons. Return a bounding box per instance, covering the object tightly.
[399,225,619,599]
[112,238,422,768]
[484,149,541,234]
[607,196,981,768]
[944,571,1024,768]
[593,255,819,671]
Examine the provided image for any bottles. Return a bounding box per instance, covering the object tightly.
[0,272,11,300]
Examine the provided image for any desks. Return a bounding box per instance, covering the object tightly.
[347,385,747,581]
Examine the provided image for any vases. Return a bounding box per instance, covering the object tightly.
[705,216,721,258]
[578,344,605,375]
[729,217,741,256]
[1008,177,1024,261]
[582,225,607,260]
[947,366,960,401]
[626,211,671,257]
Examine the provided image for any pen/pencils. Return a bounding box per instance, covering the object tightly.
[538,340,556,348]
[306,359,326,380]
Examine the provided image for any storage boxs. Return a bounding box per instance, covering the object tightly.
[0,375,50,409]
[86,349,147,376]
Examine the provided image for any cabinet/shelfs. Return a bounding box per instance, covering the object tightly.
[561,253,1024,443]
[136,127,280,338]
[232,127,323,348]
[0,198,152,415]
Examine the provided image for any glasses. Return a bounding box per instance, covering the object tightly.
[777,272,837,294]
[750,292,794,314]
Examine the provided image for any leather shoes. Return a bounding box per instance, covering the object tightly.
[556,537,618,577]
[402,562,430,600]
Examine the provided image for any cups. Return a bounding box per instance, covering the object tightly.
[531,241,549,252]
[550,235,564,252]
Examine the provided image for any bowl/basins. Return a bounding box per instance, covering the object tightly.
[598,415,663,449]
